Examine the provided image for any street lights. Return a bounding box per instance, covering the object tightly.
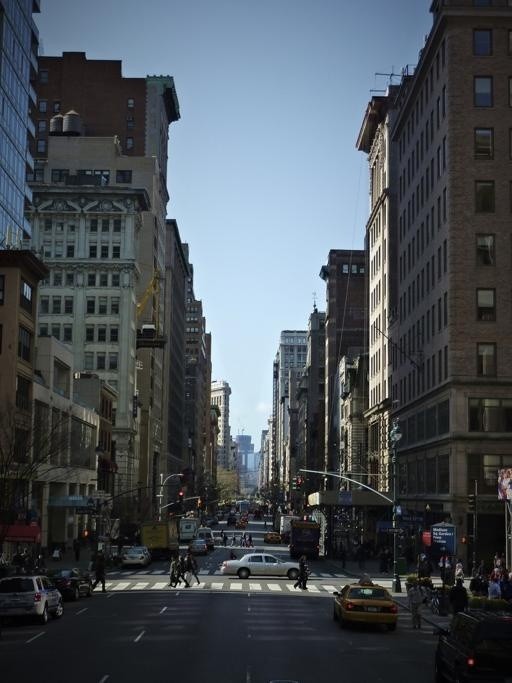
[87,497,94,536]
[389,417,402,592]
[159,474,184,520]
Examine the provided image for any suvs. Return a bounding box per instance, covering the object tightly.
[433,610,512,683]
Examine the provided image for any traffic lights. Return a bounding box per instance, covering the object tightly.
[296,477,303,487]
[468,494,476,512]
[179,491,183,501]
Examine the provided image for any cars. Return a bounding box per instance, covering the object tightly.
[228,510,248,524]
[0,568,92,625]
[221,553,300,580]
[122,546,152,568]
[180,510,224,556]
[264,531,281,544]
[333,573,398,631]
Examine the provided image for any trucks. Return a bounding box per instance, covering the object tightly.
[280,515,320,558]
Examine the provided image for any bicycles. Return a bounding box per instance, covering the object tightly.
[423,589,440,615]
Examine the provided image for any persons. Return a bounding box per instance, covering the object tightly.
[230,549,237,559]
[91,551,107,592]
[73,538,82,561]
[393,525,511,628]
[294,554,310,589]
[53,546,63,562]
[168,553,201,588]
[10,546,48,571]
[220,529,254,548]
[377,544,392,574]
[323,537,366,570]
